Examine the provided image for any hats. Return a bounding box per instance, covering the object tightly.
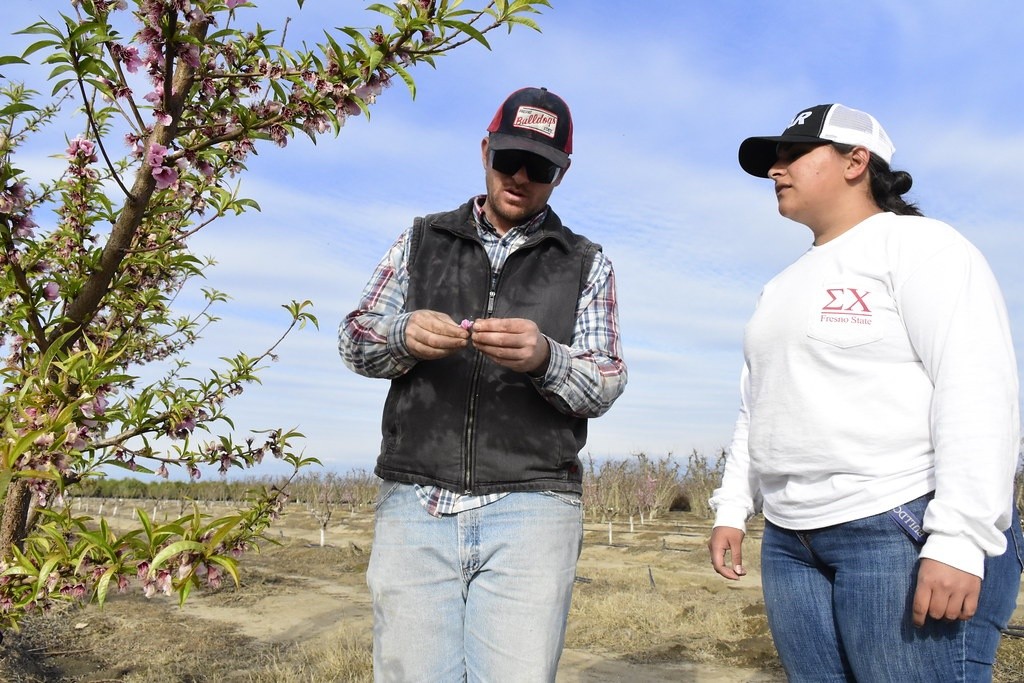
[487,87,573,169]
[738,103,894,178]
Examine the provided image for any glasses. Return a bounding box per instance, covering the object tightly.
[492,149,561,184]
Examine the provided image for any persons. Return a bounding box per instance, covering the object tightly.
[339,87,629,683]
[707,103,1024,683]
